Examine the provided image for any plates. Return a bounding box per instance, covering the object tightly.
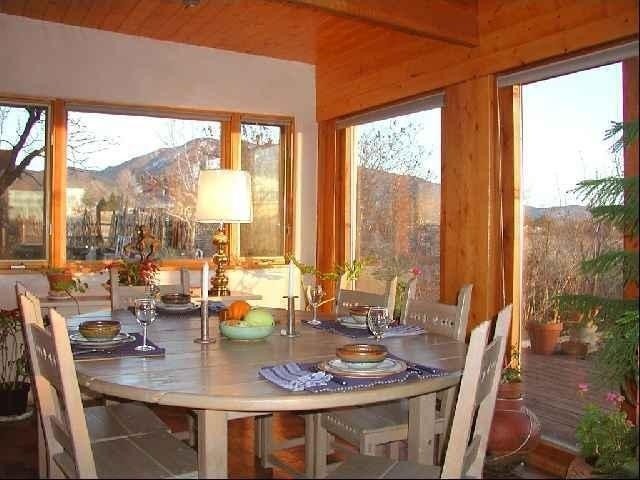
[157,302,202,314]
[339,317,395,331]
[69,330,137,350]
[323,356,408,378]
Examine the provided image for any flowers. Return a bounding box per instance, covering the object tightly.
[0,310,31,388]
[104,257,162,287]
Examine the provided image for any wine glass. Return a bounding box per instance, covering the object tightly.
[366,306,389,345]
[305,285,324,326]
[133,298,158,352]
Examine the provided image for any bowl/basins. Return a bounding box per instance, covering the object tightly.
[160,293,191,305]
[348,305,377,325]
[78,319,122,342]
[220,319,274,342]
[335,342,388,364]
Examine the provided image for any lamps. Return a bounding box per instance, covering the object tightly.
[192,169,253,297]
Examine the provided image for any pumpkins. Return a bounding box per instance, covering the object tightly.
[229,301,250,320]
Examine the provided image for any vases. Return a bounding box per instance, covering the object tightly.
[0,381,30,416]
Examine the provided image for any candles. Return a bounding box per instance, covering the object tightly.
[202,262,209,300]
[288,259,294,296]
